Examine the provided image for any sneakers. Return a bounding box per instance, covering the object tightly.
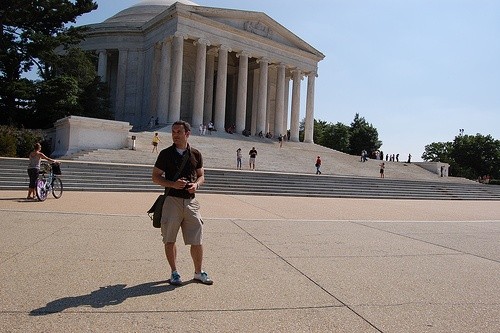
[170,271,182,286]
[194,270,213,283]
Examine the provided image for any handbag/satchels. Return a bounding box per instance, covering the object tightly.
[147,195,166,228]
[50,163,61,175]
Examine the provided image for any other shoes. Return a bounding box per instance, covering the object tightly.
[33,197,37,199]
[27,197,33,199]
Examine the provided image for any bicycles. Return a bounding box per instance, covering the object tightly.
[35,161,63,202]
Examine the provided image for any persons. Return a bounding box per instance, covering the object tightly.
[278,134,287,147]
[360,150,367,162]
[478,175,491,183]
[315,156,321,174]
[152,121,213,286]
[227,127,272,138]
[199,121,212,136]
[377,150,399,162]
[27,143,62,200]
[379,163,385,179]
[249,147,258,171]
[152,133,160,154]
[236,148,242,170]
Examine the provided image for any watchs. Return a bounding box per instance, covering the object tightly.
[196,181,199,190]
